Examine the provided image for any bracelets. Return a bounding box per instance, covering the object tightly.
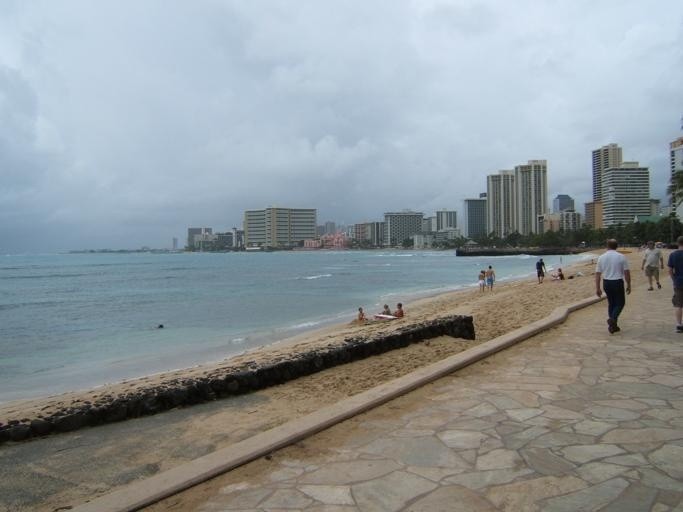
[625,287,631,290]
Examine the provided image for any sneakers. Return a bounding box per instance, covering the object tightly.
[648,287,653,291]
[657,282,661,288]
[676,325,683,333]
[607,319,615,333]
[615,327,620,332]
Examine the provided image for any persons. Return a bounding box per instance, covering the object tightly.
[550,267,563,281]
[391,302,404,317]
[665,235,683,333]
[484,265,495,293]
[594,238,631,332]
[357,306,369,321]
[379,302,392,315]
[535,258,546,284]
[477,269,486,292]
[640,240,664,291]
